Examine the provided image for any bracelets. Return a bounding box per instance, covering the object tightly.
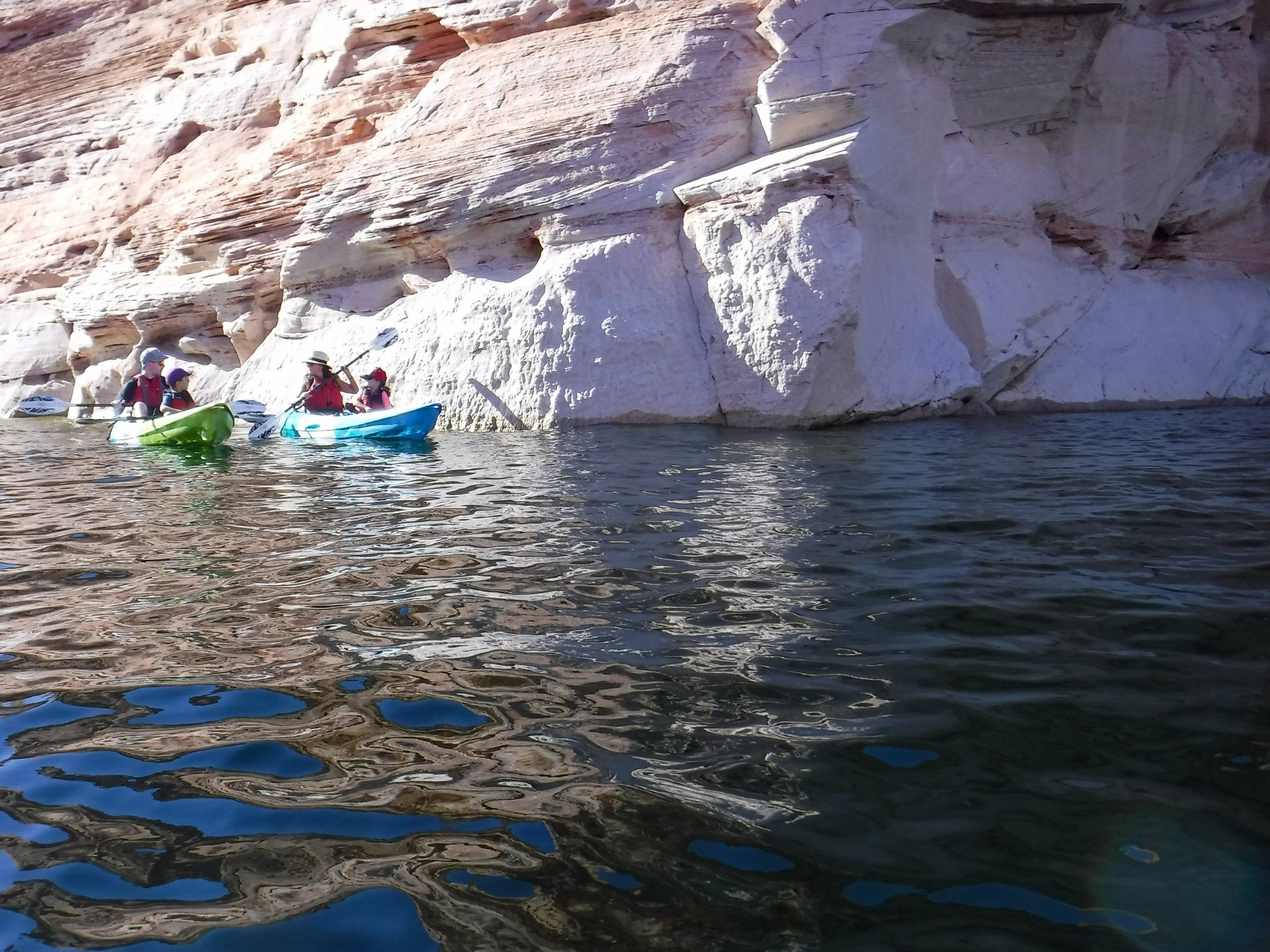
[349,377,353,380]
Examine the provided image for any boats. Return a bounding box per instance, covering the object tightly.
[278,400,443,440]
[107,401,235,447]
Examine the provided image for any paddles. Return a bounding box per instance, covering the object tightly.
[20,395,277,425]
[247,327,399,441]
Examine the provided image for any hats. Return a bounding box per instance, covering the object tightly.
[301,351,333,370]
[359,367,387,382]
[167,368,194,387]
[140,347,170,364]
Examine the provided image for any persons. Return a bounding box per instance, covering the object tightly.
[293,351,359,416]
[110,347,170,417]
[352,366,393,413]
[159,368,197,417]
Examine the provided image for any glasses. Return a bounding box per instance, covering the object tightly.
[151,359,164,366]
[308,362,314,367]
